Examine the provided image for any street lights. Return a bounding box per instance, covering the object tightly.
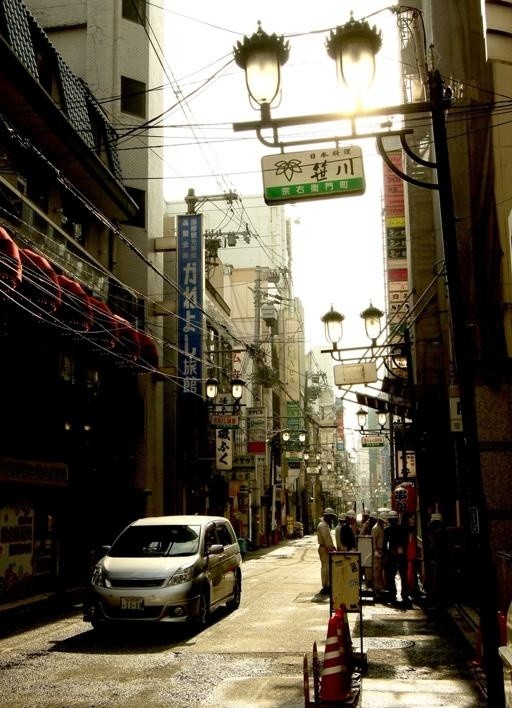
[265,429,307,546]
[303,451,331,534]
[199,373,247,512]
[232,9,506,707]
[317,303,429,578]
[355,407,397,506]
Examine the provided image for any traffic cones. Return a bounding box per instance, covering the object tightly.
[308,604,367,705]
[471,608,507,668]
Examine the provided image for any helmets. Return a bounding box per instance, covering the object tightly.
[362,508,398,523]
[323,506,337,516]
[338,510,356,521]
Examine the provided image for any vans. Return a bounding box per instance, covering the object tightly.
[84,514,242,635]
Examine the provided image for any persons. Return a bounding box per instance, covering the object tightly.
[317,507,409,604]
